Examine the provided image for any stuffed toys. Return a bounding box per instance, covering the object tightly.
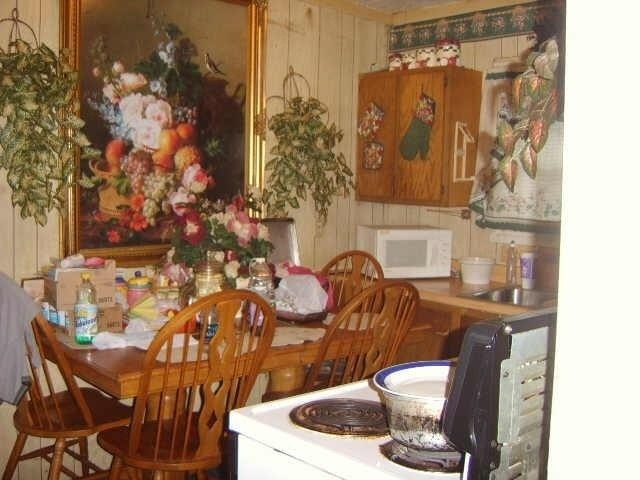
[389,38,460,71]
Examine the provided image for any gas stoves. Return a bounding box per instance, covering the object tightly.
[229,377,466,480]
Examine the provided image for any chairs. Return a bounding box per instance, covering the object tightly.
[262,278,420,402]
[1,270,133,480]
[323,249,385,316]
[96,289,277,480]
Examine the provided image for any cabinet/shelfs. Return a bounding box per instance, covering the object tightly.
[353,65,483,210]
[228,306,547,479]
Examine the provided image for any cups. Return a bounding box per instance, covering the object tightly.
[519,252,537,290]
[387,39,461,72]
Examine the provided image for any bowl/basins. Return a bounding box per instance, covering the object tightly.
[458,255,495,285]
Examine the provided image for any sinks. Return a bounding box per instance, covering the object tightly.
[454,281,559,308]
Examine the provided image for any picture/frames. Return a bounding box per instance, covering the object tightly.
[57,0,270,269]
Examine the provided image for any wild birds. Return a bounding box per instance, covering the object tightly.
[203,52,226,76]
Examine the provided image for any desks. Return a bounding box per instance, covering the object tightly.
[292,275,557,389]
[40,308,453,480]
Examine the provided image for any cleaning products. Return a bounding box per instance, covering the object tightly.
[75,274,99,345]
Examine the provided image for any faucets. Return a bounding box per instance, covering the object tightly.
[505,245,516,281]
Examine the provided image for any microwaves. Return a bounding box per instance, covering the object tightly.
[355,224,452,281]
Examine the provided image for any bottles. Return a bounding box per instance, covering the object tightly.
[506,240,518,288]
[115,245,274,344]
[73,273,99,345]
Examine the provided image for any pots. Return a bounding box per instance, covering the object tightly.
[373,359,457,451]
